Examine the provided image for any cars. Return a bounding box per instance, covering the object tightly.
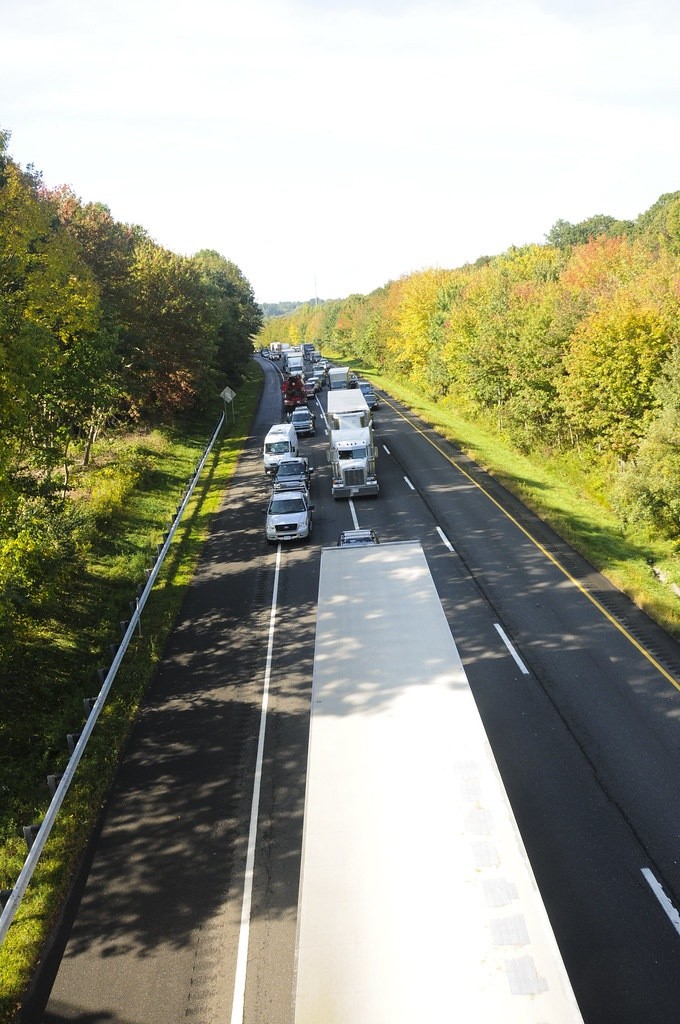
[251,341,374,408]
[294,406,310,413]
[364,394,379,410]
[260,489,314,544]
[335,530,380,549]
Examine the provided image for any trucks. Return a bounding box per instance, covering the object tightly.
[317,388,380,498]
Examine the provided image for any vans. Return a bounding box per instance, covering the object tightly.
[262,424,299,475]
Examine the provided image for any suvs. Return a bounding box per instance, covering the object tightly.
[290,411,316,436]
[265,456,313,498]
